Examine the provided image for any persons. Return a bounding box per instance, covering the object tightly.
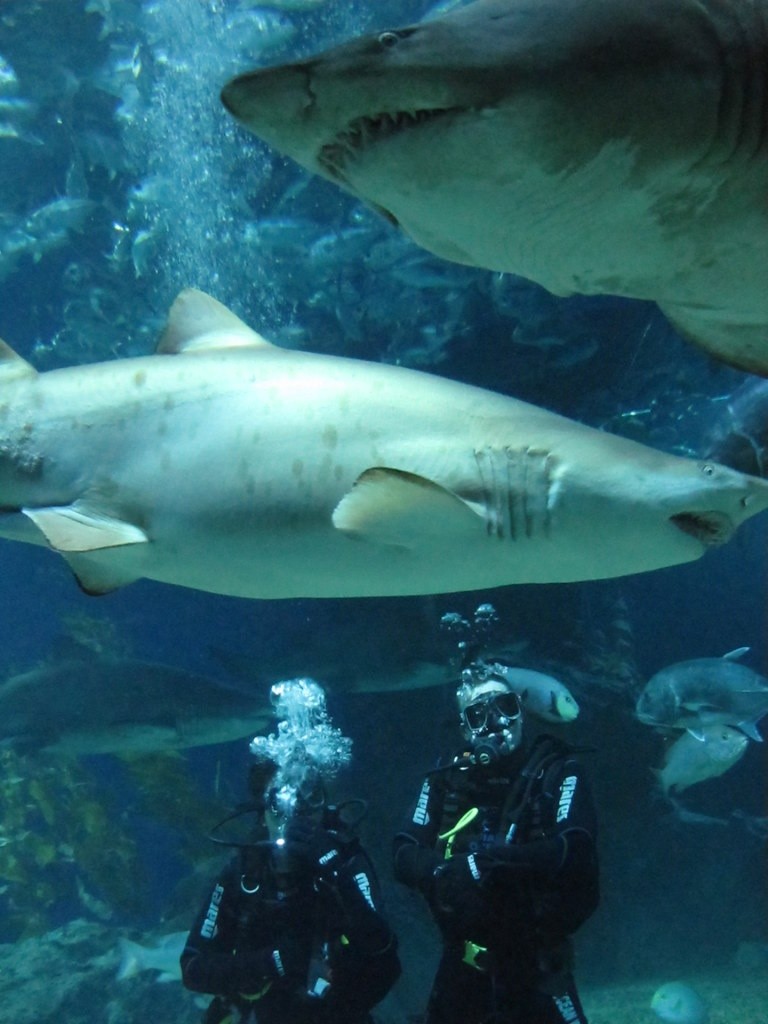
[388,662,601,1024]
[180,761,405,1024]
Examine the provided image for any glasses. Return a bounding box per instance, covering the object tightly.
[463,691,522,731]
[261,782,328,816]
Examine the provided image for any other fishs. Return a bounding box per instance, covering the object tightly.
[0,630,768,1024]
